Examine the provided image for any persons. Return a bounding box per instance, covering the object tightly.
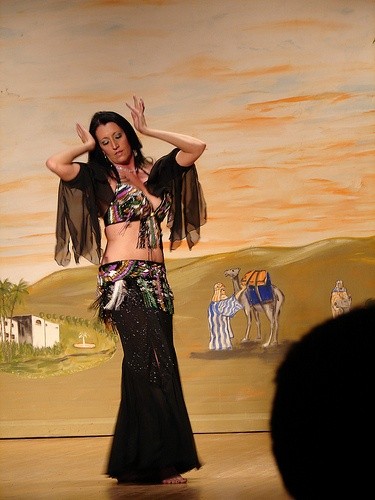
[46,96,208,485]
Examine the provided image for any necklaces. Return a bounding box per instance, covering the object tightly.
[111,162,139,172]
